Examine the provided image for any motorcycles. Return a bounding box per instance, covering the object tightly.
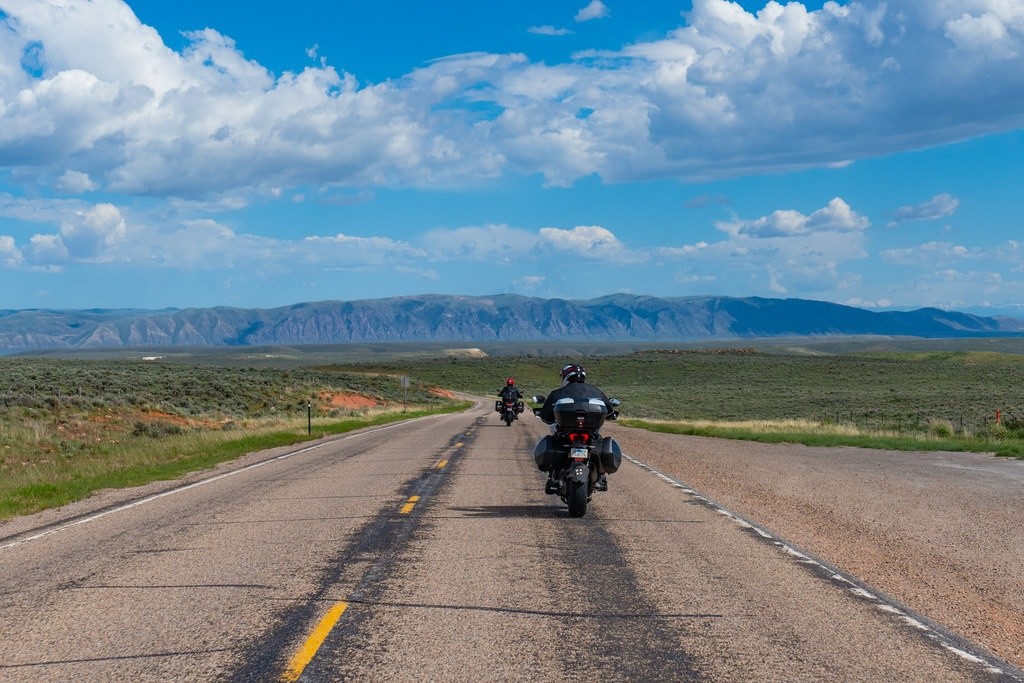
[531,382,622,518]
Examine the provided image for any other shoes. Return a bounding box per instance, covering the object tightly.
[545,479,559,495]
[594,477,608,491]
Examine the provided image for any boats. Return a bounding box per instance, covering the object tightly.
[495,388,524,426]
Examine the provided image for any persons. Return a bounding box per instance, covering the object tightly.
[498,378,523,420]
[539,363,615,495]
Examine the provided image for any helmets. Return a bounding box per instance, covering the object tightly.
[559,362,587,388]
[507,378,514,384]
